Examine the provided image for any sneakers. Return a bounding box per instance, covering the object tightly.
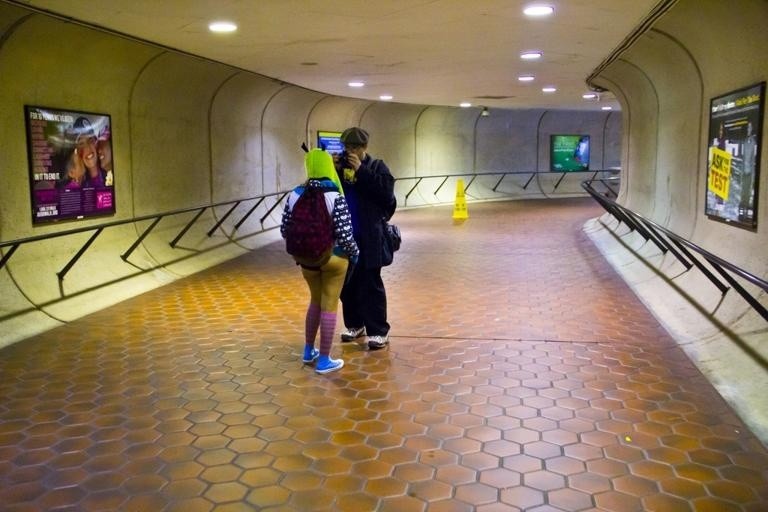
[303,347,319,363]
[340,326,366,342]
[367,331,390,348]
[316,356,344,374]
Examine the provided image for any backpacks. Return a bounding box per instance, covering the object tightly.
[286,180,337,258]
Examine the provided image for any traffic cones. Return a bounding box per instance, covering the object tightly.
[449,177,471,220]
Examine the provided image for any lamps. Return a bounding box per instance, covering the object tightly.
[481,107,490,117]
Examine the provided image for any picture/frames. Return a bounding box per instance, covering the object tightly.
[23,104,116,225]
[316,129,345,155]
[704,81,766,234]
[550,134,591,171]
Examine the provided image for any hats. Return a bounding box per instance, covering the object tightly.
[96,126,110,145]
[339,127,369,144]
[73,116,97,144]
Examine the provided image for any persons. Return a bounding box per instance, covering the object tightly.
[331,126,400,349]
[280,143,361,375]
[710,122,757,221]
[43,116,112,189]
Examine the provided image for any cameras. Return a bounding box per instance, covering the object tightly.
[339,154,352,168]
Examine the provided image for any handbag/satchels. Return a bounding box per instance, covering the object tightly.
[373,219,401,266]
